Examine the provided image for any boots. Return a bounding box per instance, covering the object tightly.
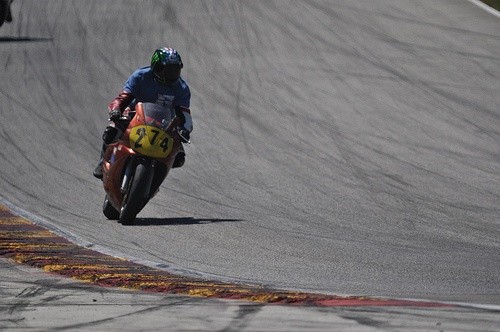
[93,142,109,178]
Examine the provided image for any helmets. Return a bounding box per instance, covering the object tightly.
[151,47,183,85]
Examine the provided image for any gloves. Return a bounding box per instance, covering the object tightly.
[109,110,121,121]
[179,130,190,143]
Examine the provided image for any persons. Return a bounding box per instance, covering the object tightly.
[93,47,191,179]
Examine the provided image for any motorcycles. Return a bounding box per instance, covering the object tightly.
[93,98,191,226]
